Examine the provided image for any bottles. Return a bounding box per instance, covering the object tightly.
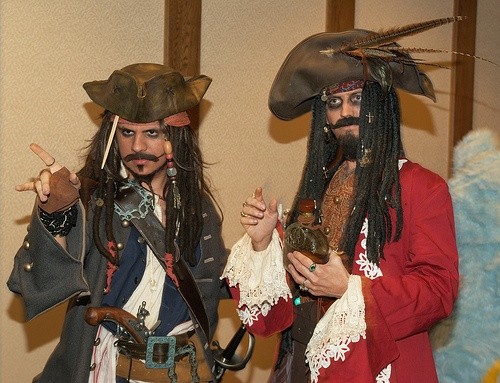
[284,198,330,275]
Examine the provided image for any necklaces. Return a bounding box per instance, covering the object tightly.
[141,185,166,201]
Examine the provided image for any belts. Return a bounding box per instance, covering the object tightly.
[118,335,189,371]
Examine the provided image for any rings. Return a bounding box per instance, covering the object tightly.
[310,263,316,272]
[243,202,246,207]
[299,278,308,290]
[241,211,247,217]
[252,193,255,198]
[46,158,55,166]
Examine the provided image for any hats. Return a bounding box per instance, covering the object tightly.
[82,62,213,128]
[267,16,500,122]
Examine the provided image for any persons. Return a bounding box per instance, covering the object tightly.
[6,62,256,383]
[218,16,500,383]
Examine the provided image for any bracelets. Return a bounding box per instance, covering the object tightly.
[38,204,78,237]
